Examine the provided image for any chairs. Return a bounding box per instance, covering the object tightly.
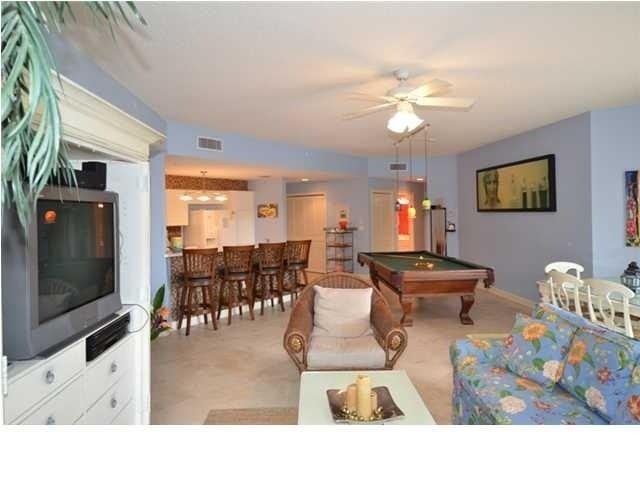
[178,247,218,336]
[278,240,312,304]
[284,271,408,375]
[218,245,254,326]
[534,262,635,339]
[252,243,286,315]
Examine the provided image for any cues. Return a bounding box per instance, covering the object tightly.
[373,254,443,262]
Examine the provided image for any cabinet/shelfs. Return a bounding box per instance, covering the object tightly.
[323,228,358,273]
[1,307,152,425]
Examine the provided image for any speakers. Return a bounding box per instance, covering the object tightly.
[82,162,106,189]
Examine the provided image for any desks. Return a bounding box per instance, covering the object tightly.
[357,250,494,325]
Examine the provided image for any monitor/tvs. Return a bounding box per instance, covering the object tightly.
[1,182,123,360]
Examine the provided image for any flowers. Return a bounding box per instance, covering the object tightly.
[151,283,173,343]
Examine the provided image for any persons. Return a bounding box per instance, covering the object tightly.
[483,169,502,208]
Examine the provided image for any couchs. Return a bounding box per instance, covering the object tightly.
[448,303,640,425]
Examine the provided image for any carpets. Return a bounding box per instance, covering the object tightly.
[203,407,298,425]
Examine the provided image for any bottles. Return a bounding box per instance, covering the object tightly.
[343,374,379,419]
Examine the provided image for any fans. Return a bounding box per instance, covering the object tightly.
[343,69,477,120]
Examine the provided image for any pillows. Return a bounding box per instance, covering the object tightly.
[609,351,640,424]
[558,323,640,423]
[532,302,589,330]
[312,284,374,340]
[495,311,578,393]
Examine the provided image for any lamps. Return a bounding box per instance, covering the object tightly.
[386,91,424,134]
[179,170,230,203]
[388,122,431,219]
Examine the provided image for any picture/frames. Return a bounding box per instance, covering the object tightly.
[257,204,278,217]
[476,154,556,212]
